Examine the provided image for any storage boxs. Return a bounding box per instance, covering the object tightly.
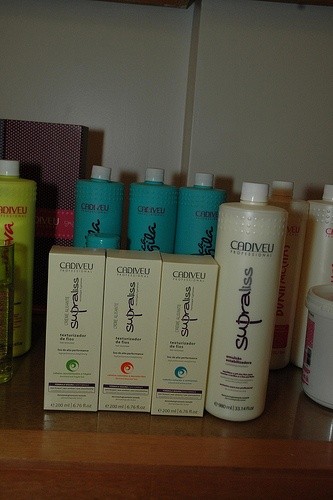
[4,118,89,333]
[150,254,221,417]
[42,247,107,413]
[97,250,162,412]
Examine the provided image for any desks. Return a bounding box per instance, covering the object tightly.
[0,349,333,500]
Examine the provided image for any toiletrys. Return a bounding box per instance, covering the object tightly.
[264,179,307,370]
[0,159,37,356]
[127,168,177,255]
[204,181,289,423]
[72,164,121,249]
[291,182,333,368]
[179,171,227,258]
